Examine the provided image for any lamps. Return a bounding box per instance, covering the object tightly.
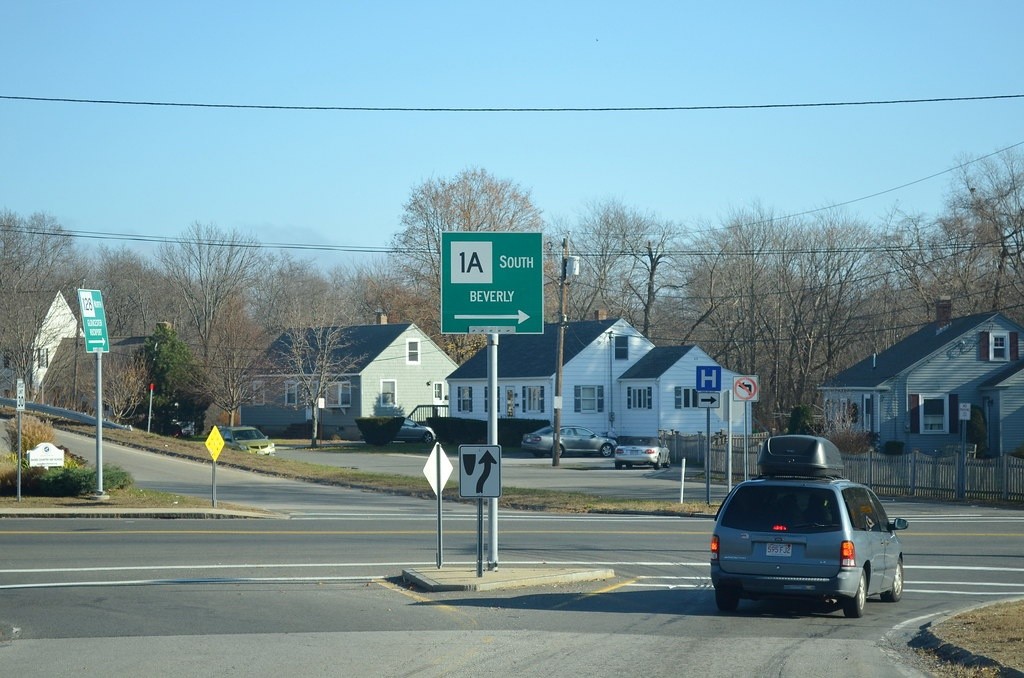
[987,399,994,406]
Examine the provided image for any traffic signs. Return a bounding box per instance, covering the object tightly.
[79,289,110,354]
[439,231,545,335]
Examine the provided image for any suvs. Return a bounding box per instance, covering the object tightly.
[710,434,909,619]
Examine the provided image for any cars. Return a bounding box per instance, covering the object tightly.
[614,435,670,470]
[392,417,437,444]
[217,426,276,457]
[520,424,618,459]
